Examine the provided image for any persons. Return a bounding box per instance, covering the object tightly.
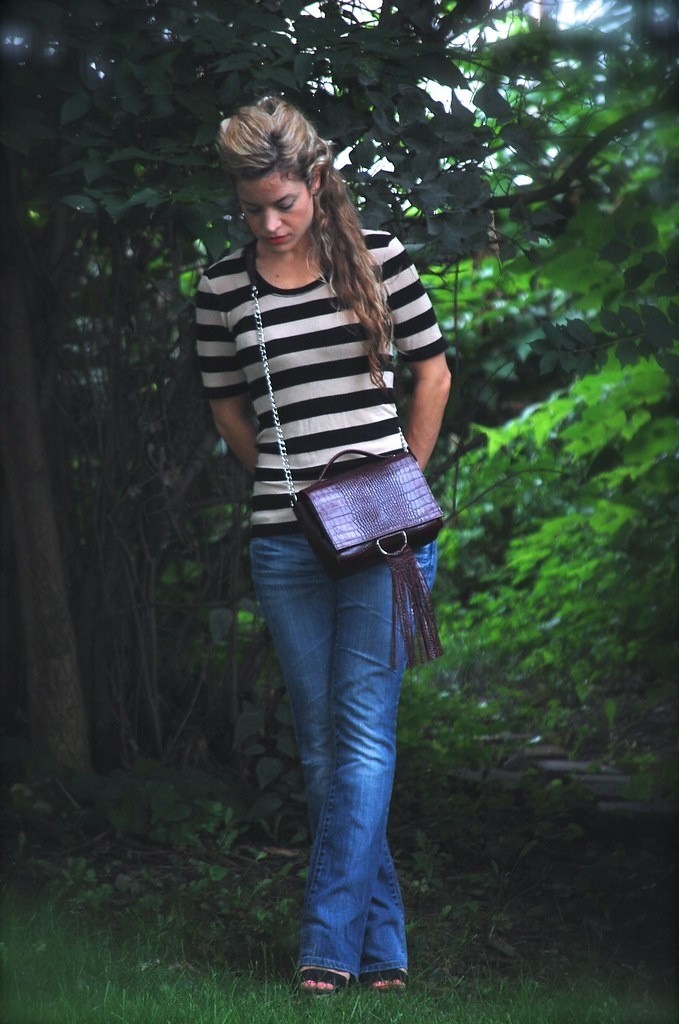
[193,95,451,996]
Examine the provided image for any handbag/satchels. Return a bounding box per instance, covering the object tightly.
[291,449,445,669]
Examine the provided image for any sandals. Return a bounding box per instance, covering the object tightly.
[360,969,409,993]
[298,969,347,998]
